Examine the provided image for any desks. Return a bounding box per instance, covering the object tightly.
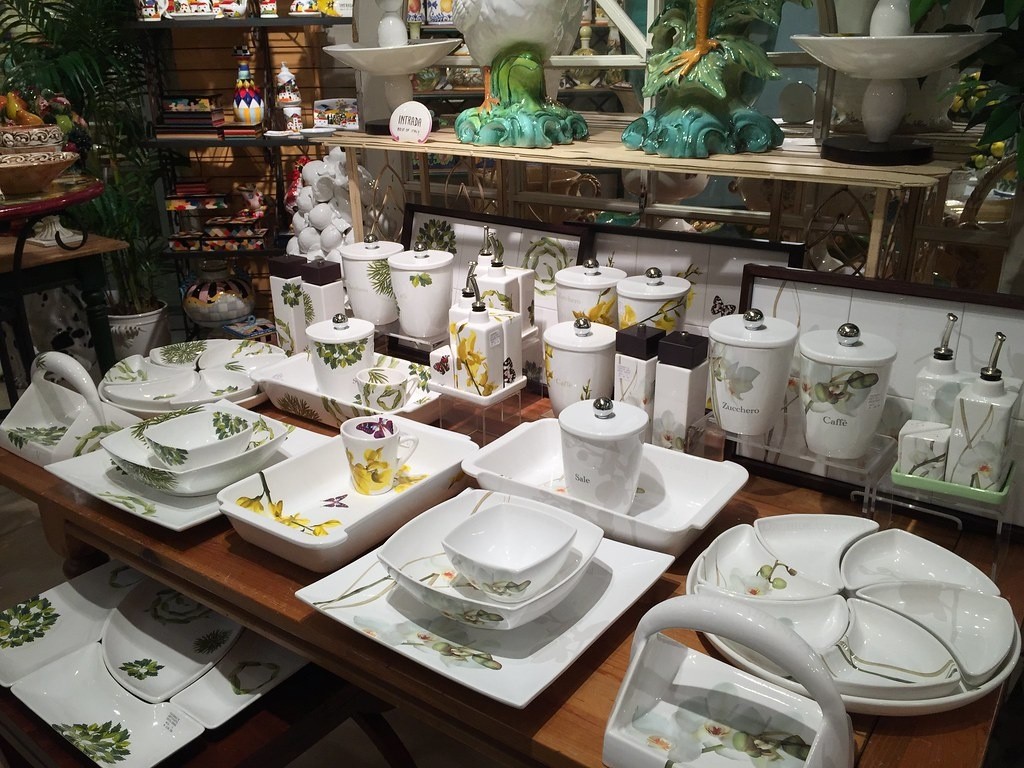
[0,228,130,419]
[306,108,961,278]
[1,337,1024,768]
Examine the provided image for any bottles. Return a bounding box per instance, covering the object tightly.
[231,54,264,126]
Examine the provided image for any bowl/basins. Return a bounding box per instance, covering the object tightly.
[791,31,1003,78]
[375,489,606,631]
[322,36,462,76]
[0,123,81,197]
[99,403,289,498]
[144,410,253,473]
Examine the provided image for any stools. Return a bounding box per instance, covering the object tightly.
[1,662,417,768]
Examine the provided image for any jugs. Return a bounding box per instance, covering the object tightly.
[568,37,603,90]
[406,21,440,92]
[182,265,256,328]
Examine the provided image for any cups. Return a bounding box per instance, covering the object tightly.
[388,242,454,339]
[355,367,421,413]
[304,313,376,402]
[342,234,404,325]
[558,397,650,514]
[708,308,798,436]
[554,258,629,332]
[617,267,691,335]
[542,317,620,418]
[798,323,898,460]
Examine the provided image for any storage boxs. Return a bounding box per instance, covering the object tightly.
[168,230,203,251]
[201,229,271,251]
[202,216,261,237]
[165,194,231,210]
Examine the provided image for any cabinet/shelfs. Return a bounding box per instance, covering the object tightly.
[406,19,627,199]
[119,17,353,260]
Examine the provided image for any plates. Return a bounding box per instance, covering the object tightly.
[250,351,455,429]
[170,12,217,21]
[294,487,677,709]
[298,127,336,138]
[461,419,750,562]
[99,337,288,420]
[683,512,1022,715]
[44,398,335,532]
[0,557,325,768]
[288,11,323,17]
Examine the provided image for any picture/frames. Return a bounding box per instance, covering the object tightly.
[386,202,597,398]
[722,263,1024,544]
[561,219,806,415]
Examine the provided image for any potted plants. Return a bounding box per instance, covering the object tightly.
[0,0,172,359]
